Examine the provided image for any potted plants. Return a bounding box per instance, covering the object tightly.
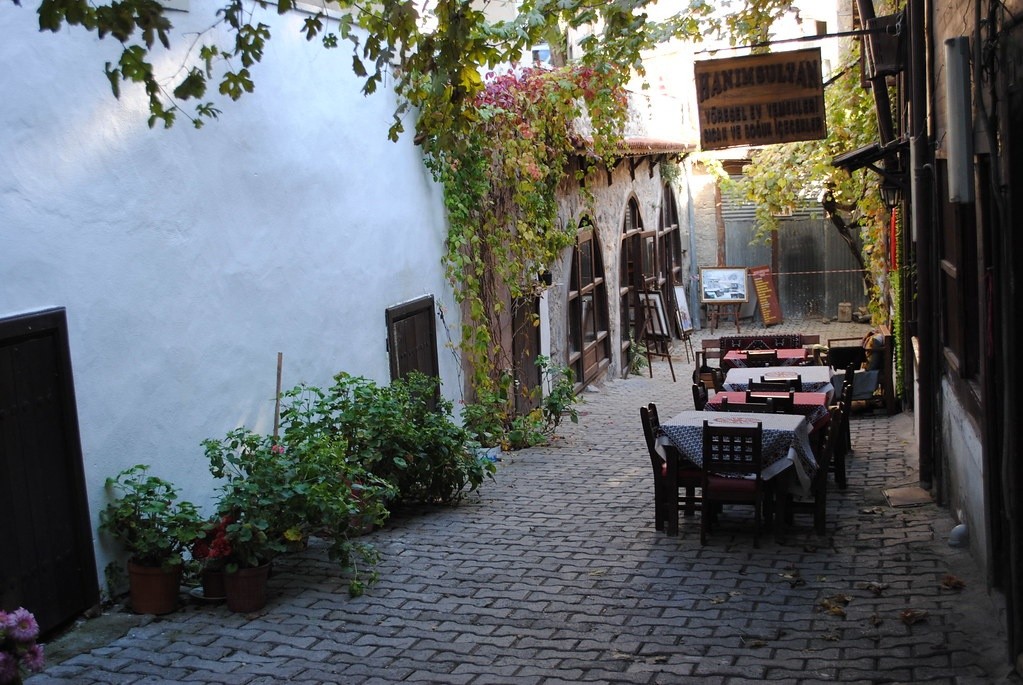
[215,517,286,614]
[96,464,217,615]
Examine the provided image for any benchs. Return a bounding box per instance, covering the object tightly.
[701,334,821,389]
[827,324,896,416]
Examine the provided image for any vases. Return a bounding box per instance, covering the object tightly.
[198,571,226,598]
[351,515,374,537]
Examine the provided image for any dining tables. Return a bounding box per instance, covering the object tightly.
[721,366,835,407]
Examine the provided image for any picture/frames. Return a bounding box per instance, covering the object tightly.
[637,290,673,342]
[671,283,695,335]
[699,266,749,303]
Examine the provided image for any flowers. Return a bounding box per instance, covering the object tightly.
[338,476,373,515]
[192,513,232,569]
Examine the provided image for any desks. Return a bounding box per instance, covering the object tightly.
[703,392,829,485]
[721,349,807,368]
[654,411,820,538]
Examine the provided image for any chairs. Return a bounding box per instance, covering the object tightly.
[639,348,855,549]
